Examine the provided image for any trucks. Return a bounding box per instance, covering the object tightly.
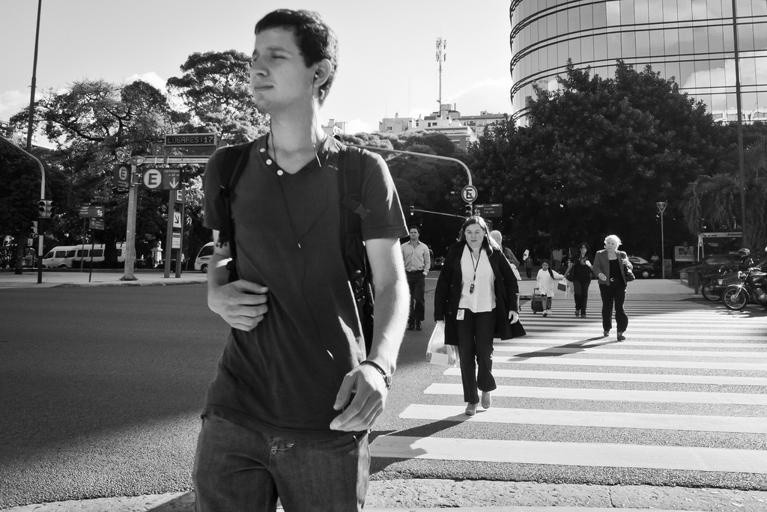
[41,245,77,269]
[57,241,136,269]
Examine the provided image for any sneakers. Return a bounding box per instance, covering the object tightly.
[482,392,490,409]
[466,402,479,416]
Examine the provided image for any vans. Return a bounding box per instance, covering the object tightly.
[194,242,215,273]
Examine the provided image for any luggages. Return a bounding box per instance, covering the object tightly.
[531,288,543,314]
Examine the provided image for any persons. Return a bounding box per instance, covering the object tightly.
[187,8,412,512]
[488,228,520,286]
[565,240,594,318]
[401,222,431,337]
[591,233,635,343]
[433,216,528,417]
[536,258,564,318]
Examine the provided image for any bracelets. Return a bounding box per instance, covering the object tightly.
[356,358,393,393]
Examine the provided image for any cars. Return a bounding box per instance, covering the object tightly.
[431,258,444,270]
[627,256,660,279]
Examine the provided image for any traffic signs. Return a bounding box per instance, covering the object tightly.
[475,203,502,217]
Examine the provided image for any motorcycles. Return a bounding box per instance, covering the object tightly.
[721,258,767,311]
[702,259,761,302]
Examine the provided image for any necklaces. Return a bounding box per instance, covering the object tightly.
[468,251,481,295]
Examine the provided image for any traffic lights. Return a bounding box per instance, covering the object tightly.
[30,221,37,235]
[333,134,343,146]
[464,205,472,222]
[409,206,414,216]
[47,200,55,218]
[38,200,46,218]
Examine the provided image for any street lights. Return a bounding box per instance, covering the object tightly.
[657,202,667,279]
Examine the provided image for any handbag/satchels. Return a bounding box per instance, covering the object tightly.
[558,281,570,292]
[624,266,636,281]
